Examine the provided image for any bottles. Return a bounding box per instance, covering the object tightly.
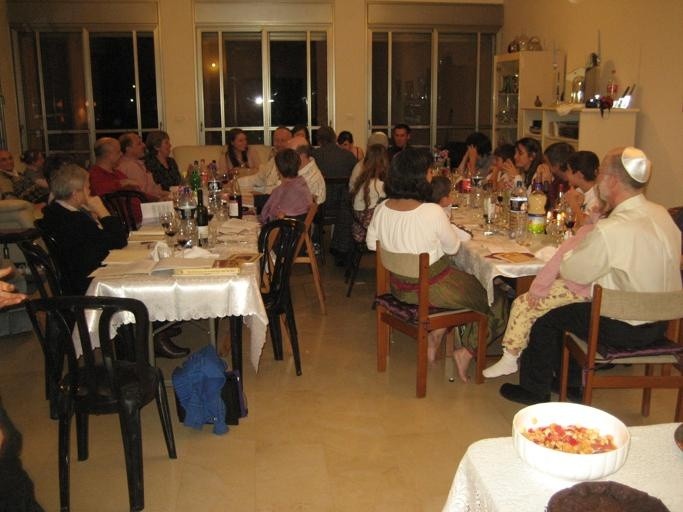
[534,95,542,106]
[607,69,618,101]
[432,151,586,247]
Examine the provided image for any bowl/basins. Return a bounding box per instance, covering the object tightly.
[510,399,632,480]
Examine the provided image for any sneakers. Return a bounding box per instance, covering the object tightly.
[330,247,348,267]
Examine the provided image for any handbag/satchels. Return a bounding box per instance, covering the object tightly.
[175,369,247,425]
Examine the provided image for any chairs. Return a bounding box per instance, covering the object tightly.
[442,139,467,169]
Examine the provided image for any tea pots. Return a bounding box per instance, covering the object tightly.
[504,36,542,53]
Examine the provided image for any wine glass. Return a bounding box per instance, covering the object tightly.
[157,156,243,262]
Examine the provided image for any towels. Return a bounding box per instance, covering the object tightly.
[556,103,585,117]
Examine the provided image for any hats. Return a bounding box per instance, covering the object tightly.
[621,146,651,183]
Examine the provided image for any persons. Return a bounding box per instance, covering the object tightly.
[0,266,46,512]
[1,123,683,407]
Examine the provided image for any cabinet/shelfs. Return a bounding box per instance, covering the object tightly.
[520,106,640,165]
[491,49,553,155]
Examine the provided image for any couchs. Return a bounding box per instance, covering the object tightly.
[171,144,271,178]
[0,160,50,268]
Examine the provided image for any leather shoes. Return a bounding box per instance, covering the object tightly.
[155,328,190,359]
[551,376,583,403]
[500,382,551,405]
